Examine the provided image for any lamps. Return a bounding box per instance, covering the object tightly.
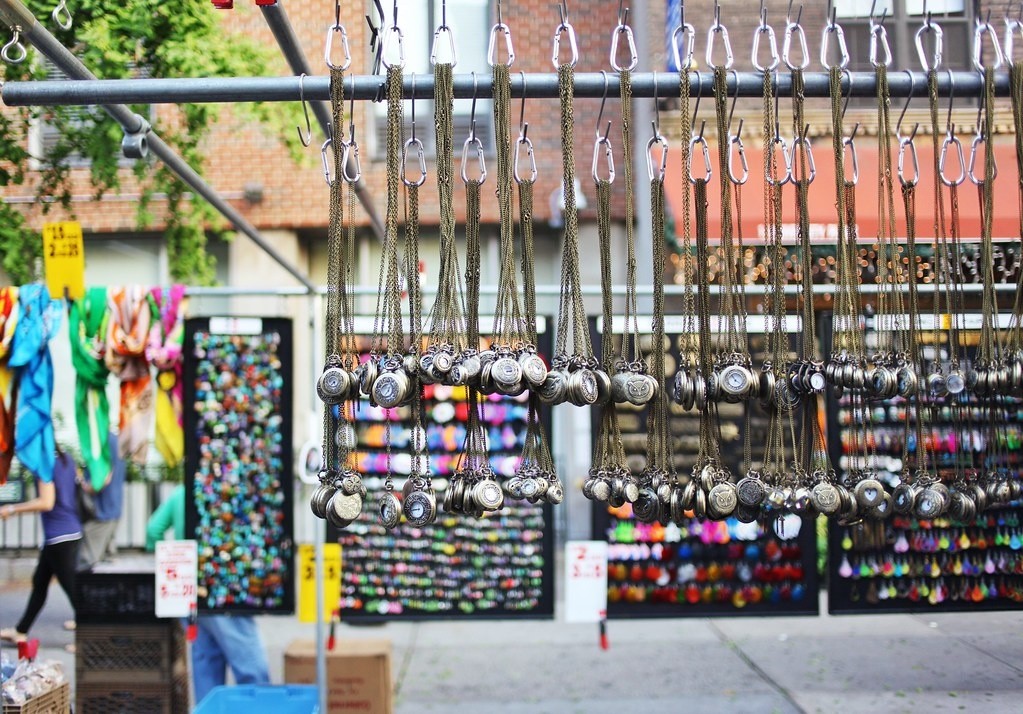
[549,177,588,230]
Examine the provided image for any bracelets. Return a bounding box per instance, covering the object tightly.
[9,505,14,516]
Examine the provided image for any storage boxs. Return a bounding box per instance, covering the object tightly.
[191,683,320,714]
[283,639,394,714]
[1,680,69,714]
[76,673,191,714]
[74,624,186,680]
[72,568,179,623]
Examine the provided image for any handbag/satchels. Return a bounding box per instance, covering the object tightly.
[75,482,97,524]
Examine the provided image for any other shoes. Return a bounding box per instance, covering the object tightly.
[63,620,76,630]
[0,628,27,644]
[65,643,76,653]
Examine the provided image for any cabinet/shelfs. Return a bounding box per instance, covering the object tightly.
[588,314,819,618]
[326,312,555,625]
[817,310,1023,615]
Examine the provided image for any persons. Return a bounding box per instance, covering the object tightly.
[145,484,271,703]
[64,431,123,629]
[0,435,85,653]
